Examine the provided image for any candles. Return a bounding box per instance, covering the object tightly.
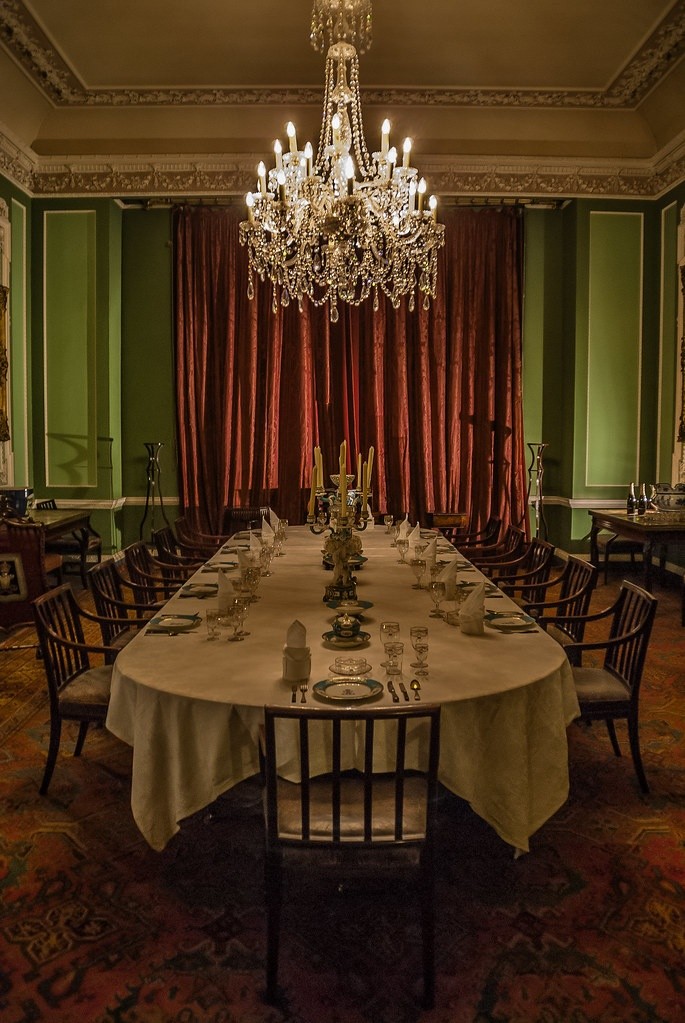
[307,440,374,517]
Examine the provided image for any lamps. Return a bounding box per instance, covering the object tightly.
[239,0,445,326]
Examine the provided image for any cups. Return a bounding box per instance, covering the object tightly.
[384,642,404,676]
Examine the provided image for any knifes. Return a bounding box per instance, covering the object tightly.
[388,681,409,703]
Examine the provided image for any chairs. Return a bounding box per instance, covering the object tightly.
[33,515,235,795]
[438,512,658,792]
[255,704,444,1003]
[0,516,65,659]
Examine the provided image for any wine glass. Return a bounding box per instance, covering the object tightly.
[384,515,470,626]
[379,621,430,677]
[206,519,289,642]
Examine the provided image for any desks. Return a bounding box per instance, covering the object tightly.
[587,507,685,591]
[2,508,90,597]
[106,516,580,864]
[38,495,179,508]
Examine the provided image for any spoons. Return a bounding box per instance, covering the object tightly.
[485,609,520,615]
[496,626,539,634]
[292,680,298,702]
[410,679,421,700]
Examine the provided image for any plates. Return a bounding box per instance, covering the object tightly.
[419,532,537,627]
[313,676,384,700]
[149,532,262,628]
[321,555,374,676]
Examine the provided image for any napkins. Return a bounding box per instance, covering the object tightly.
[396,513,485,616]
[217,507,282,609]
[286,619,306,650]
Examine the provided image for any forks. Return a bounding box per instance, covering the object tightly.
[301,678,308,702]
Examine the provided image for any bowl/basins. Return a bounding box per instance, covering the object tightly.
[649,482,685,511]
[335,657,367,673]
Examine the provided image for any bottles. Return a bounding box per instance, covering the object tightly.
[627,482,647,514]
[332,613,361,641]
